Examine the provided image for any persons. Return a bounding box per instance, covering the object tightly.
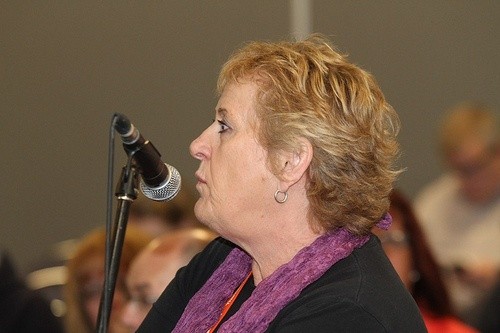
[131,33,430,333]
[128,181,201,242]
[67,222,153,333]
[0,246,63,333]
[370,187,479,332]
[121,228,220,332]
[409,99,499,333]
